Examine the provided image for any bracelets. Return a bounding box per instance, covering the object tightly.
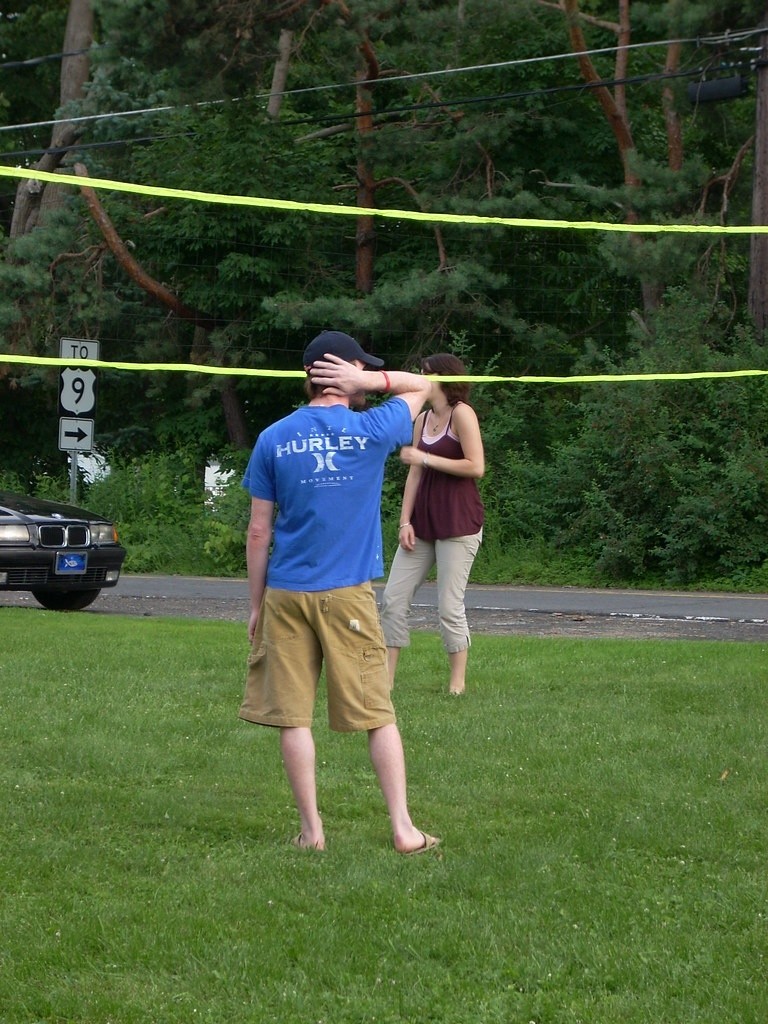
[398,522,410,531]
[379,370,390,394]
[422,452,429,468]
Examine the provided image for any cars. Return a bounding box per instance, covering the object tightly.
[0,490,127,612]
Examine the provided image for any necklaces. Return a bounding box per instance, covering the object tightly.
[431,406,447,432]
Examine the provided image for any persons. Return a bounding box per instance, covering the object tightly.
[236,331,443,855]
[380,353,485,696]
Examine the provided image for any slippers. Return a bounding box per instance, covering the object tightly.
[406,831,440,856]
[290,832,303,847]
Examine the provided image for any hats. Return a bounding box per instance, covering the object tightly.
[303,330,385,367]
